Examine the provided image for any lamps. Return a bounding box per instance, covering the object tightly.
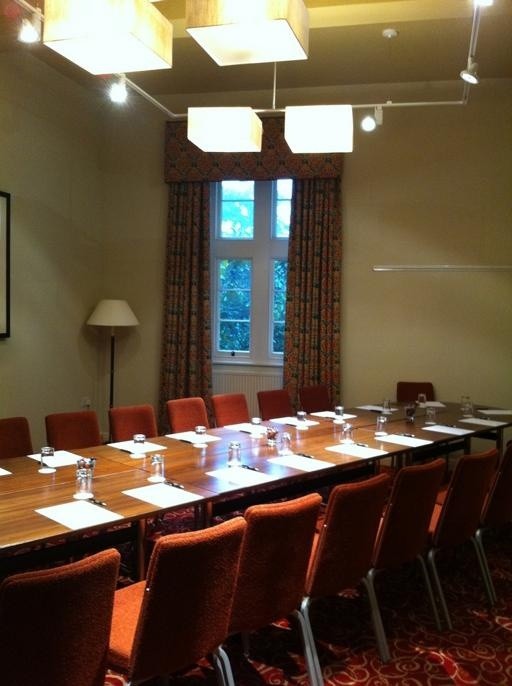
[18,7,42,43]
[284,104,355,153]
[460,55,479,86]
[79,296,142,442]
[108,76,128,104]
[186,1,310,67]
[187,107,262,153]
[362,107,383,132]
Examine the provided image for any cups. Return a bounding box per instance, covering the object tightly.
[252,417,261,436]
[382,398,392,415]
[149,454,165,481]
[377,416,387,433]
[194,426,208,448]
[133,434,145,456]
[335,406,344,422]
[40,447,55,472]
[276,434,290,456]
[343,424,354,446]
[226,441,242,466]
[77,459,94,496]
[265,423,281,447]
[406,393,473,425]
[296,411,306,429]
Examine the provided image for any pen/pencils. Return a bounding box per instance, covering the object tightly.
[446,424,456,427]
[120,449,134,454]
[240,431,250,434]
[241,465,259,471]
[165,481,184,489]
[356,444,368,447]
[370,409,381,414]
[296,453,312,458]
[39,462,47,465]
[476,408,488,410]
[89,498,107,506]
[286,424,296,427]
[179,440,191,444]
[403,434,416,437]
[480,418,490,419]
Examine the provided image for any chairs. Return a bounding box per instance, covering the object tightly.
[0,379,512,685]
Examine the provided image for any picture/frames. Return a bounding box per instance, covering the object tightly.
[0,187,12,342]
